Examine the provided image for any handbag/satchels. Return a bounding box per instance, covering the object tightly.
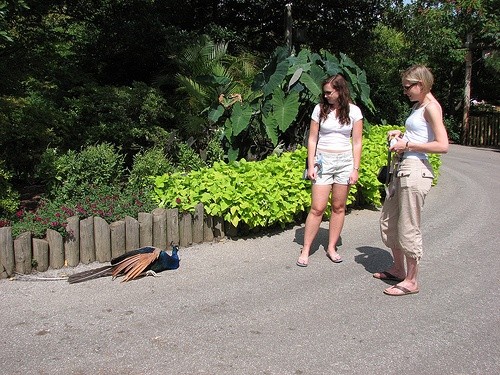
[305,155,320,180]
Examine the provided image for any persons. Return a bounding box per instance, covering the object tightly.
[373,65,449,295]
[296,72,364,267]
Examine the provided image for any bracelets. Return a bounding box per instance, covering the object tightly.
[406,141,409,152]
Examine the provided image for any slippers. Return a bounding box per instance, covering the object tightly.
[373,272,404,281]
[296,257,307,266]
[383,284,419,296]
[326,253,343,263]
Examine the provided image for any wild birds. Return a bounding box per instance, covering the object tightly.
[13,241,181,283]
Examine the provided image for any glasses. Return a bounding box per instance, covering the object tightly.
[323,91,336,97]
[401,83,417,91]
